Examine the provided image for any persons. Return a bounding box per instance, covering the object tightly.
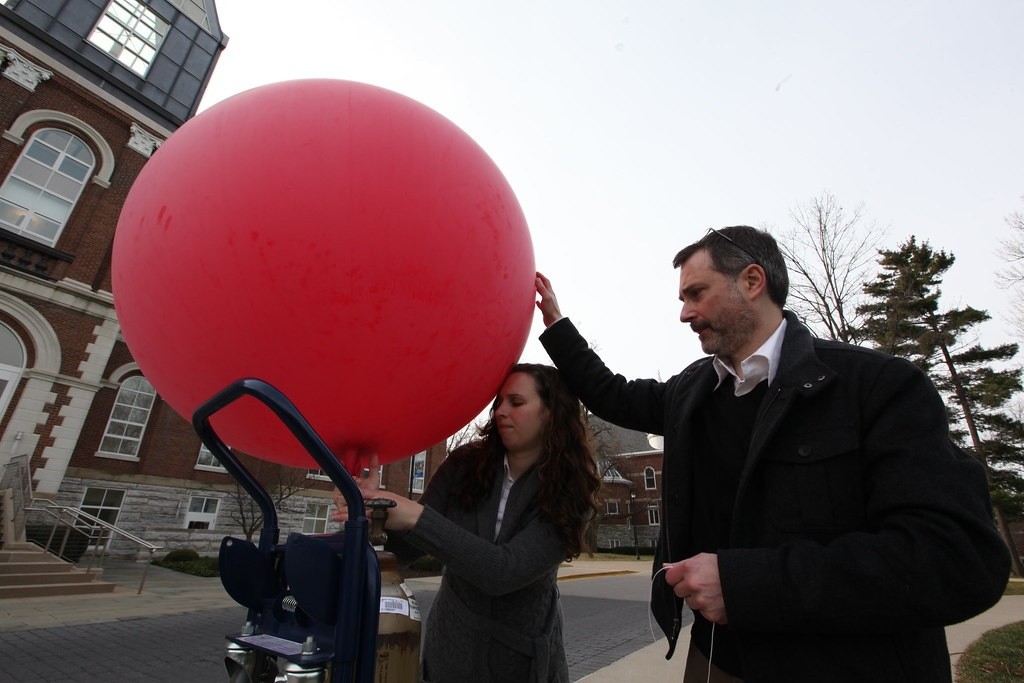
[330,365,603,683]
[534,224,1010,683]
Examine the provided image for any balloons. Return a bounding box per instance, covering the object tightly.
[111,78,537,475]
